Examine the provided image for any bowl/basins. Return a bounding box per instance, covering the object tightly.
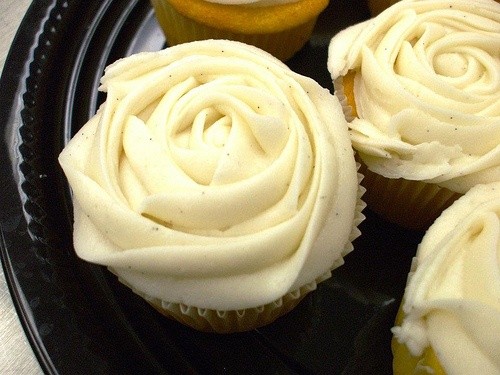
[0,0,429,375]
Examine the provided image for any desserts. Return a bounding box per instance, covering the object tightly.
[151,0,334,60]
[55,38,359,311]
[327,0,500,375]
